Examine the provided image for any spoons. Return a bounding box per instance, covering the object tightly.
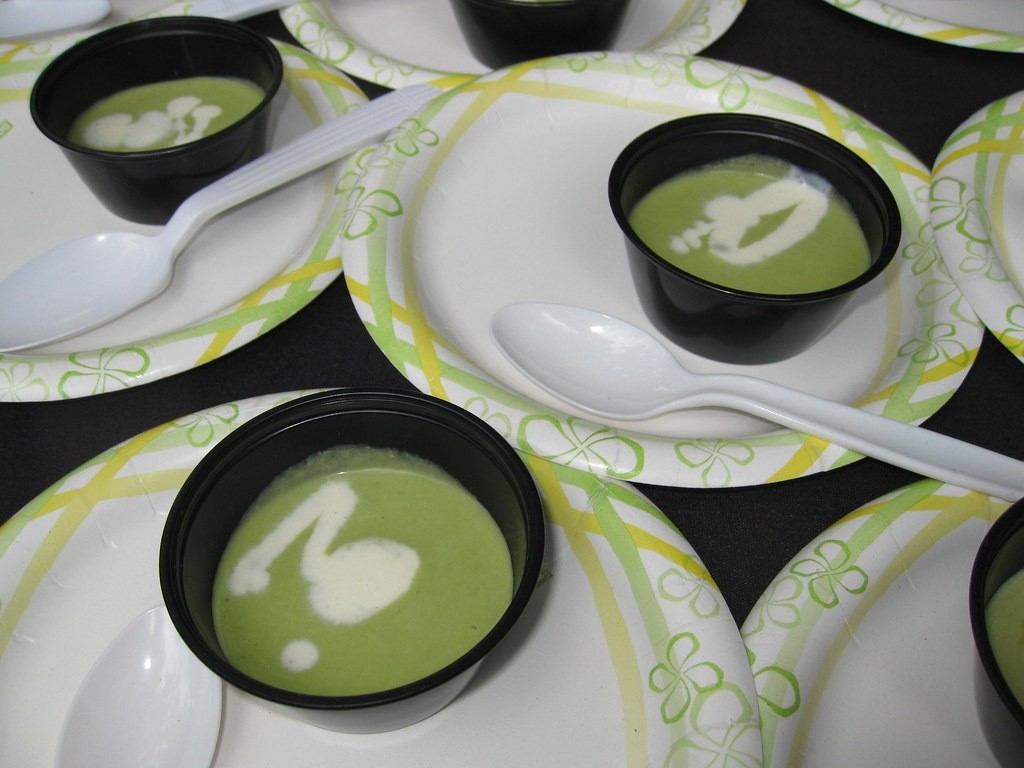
[55,604,222,768]
[0,0,112,42]
[0,84,444,351]
[488,301,1024,504]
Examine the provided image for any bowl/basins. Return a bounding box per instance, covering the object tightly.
[608,113,902,365]
[968,497,1024,730]
[29,16,284,226]
[450,0,631,71]
[159,389,546,734]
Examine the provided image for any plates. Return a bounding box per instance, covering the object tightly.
[928,91,1023,361]
[279,0,749,91]
[342,52,984,487]
[1,1,299,63]
[740,478,1024,767]
[1,388,762,768]
[1,37,372,403]
[826,1,1023,54]
